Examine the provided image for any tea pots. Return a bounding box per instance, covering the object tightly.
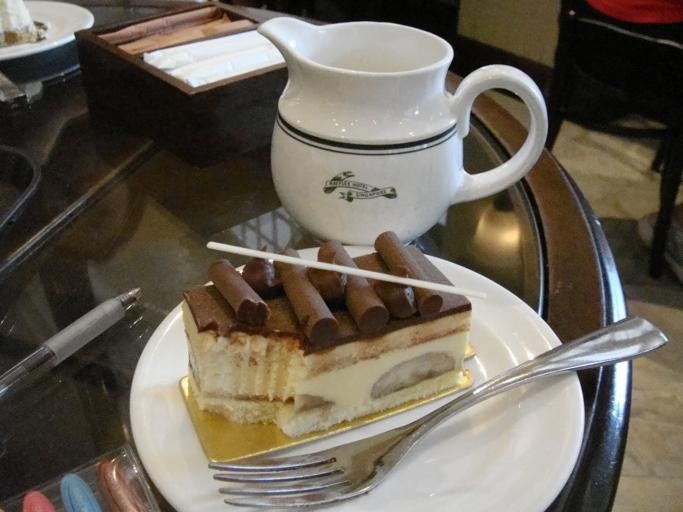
[258,15,549,248]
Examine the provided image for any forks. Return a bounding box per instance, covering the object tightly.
[208,315,667,508]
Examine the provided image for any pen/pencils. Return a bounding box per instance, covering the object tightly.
[0,287,144,405]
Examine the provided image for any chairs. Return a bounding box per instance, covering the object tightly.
[547,0,683,276]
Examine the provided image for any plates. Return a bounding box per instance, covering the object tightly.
[127,250,587,512]
[0,1,96,61]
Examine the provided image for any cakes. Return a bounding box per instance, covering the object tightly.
[181,230,470,439]
[1,0,47,50]
